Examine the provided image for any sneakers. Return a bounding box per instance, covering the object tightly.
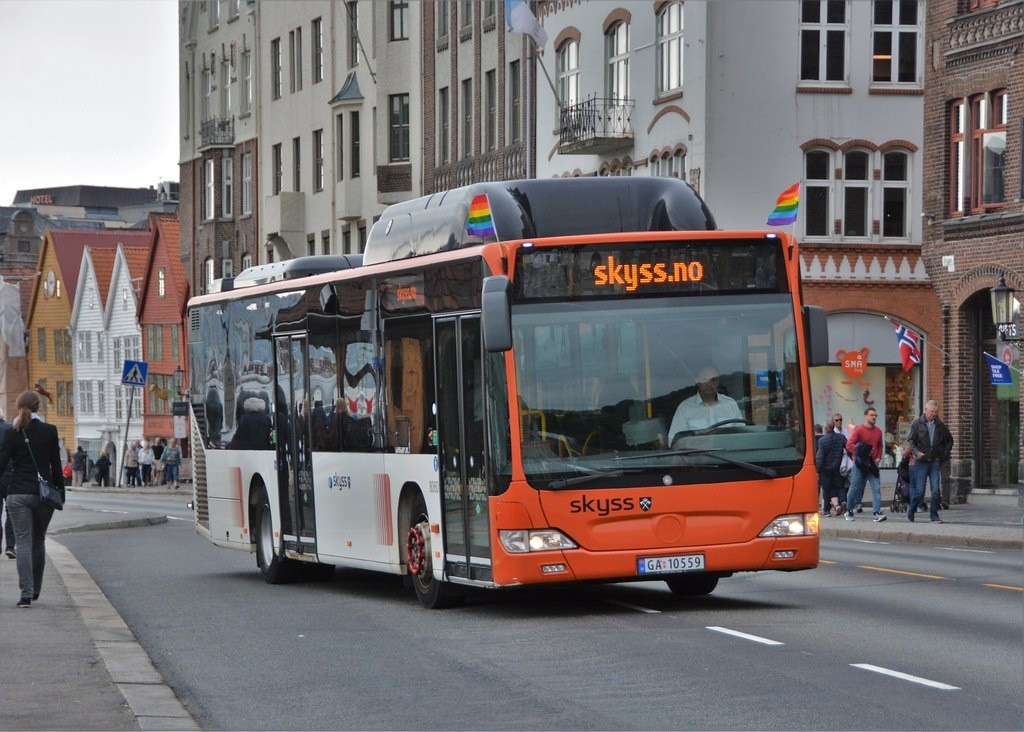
[843,509,856,521]
[872,512,887,522]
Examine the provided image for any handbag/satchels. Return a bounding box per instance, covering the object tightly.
[38,476,63,510]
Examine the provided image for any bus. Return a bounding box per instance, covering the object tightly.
[188,177,829,609]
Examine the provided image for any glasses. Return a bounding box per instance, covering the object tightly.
[834,419,842,422]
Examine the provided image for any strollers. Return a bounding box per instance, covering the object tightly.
[890,448,928,513]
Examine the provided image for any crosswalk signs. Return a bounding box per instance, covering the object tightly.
[121,360,148,386]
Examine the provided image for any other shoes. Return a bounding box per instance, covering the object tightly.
[840,502,847,515]
[819,510,831,517]
[5,548,16,559]
[930,516,943,523]
[834,505,841,516]
[907,511,914,522]
[32,594,39,600]
[16,598,31,608]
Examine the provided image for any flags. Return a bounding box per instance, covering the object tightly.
[766,181,799,226]
[889,318,923,372]
[983,352,1014,385]
[466,194,495,238]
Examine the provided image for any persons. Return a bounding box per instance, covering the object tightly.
[0,409,18,561]
[0,392,66,607]
[813,413,864,517]
[901,400,954,524]
[123,436,183,490]
[845,408,889,522]
[204,383,375,452]
[668,362,747,449]
[62,445,112,488]
